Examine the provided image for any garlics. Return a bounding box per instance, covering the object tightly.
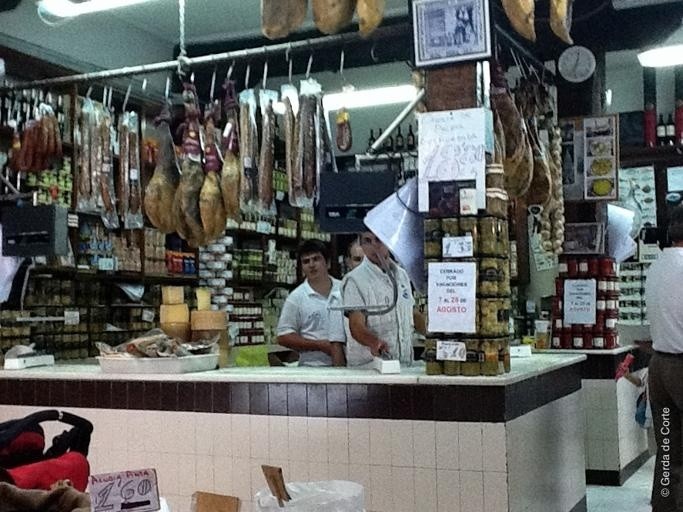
[537,124,564,263]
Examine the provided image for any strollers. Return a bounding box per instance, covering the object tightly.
[0,408,93,495]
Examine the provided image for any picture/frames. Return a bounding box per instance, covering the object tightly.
[408,0,495,69]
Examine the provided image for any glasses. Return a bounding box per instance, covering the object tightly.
[362,237,383,244]
[352,256,364,262]
[302,256,323,264]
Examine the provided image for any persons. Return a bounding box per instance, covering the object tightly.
[273,237,341,368]
[337,229,426,370]
[638,201,682,511]
[325,238,364,369]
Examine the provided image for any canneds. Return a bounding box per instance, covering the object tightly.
[25,157,73,208]
[420,215,512,375]
[0,205,331,368]
[552,256,620,348]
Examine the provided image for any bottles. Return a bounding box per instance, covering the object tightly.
[655,113,666,147]
[0,271,160,364]
[368,123,415,153]
[666,113,677,147]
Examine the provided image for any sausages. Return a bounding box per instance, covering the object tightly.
[239,93,333,208]
[80,109,139,218]
[8,112,62,173]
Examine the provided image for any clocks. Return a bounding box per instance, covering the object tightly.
[557,45,597,84]
